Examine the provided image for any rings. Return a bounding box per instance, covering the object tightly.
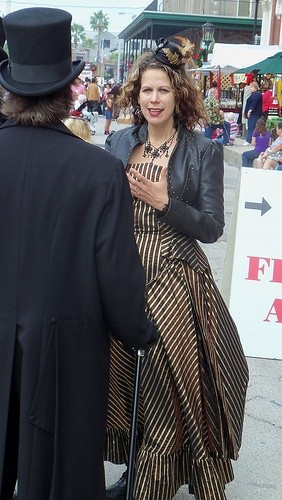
[135,188,139,192]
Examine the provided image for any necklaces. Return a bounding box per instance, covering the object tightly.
[143,128,178,163]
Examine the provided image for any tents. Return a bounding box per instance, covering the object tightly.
[187,43,282,74]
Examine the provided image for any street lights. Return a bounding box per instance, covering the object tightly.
[203,21,215,95]
[119,11,136,19]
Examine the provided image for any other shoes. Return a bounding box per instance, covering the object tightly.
[106,470,127,499]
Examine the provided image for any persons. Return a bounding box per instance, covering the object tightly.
[201,69,282,171]
[0,8,161,500]
[104,39,250,500]
[63,77,123,143]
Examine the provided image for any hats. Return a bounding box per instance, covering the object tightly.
[155,36,196,69]
[0,7,86,96]
